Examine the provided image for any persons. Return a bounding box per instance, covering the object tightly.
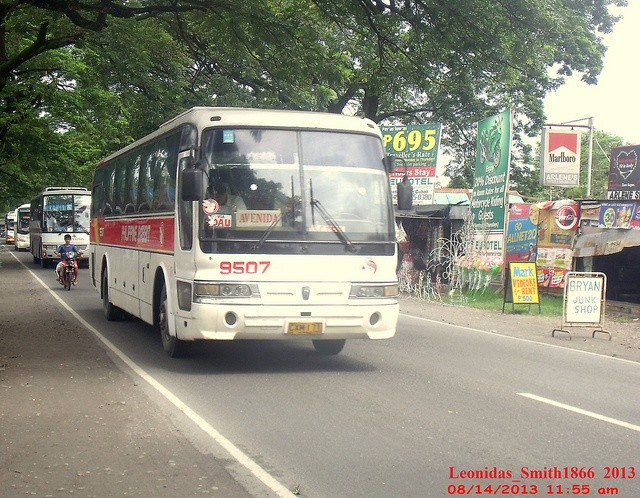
[54,233,79,286]
[205,183,247,229]
[43,211,57,232]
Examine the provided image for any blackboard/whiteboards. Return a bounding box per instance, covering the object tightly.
[567,277,603,323]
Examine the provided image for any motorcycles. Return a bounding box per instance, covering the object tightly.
[54,249,84,290]
[478,114,504,169]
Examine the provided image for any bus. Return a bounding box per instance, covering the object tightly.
[29,185,91,269]
[88,105,400,356]
[13,203,30,251]
[5,210,14,244]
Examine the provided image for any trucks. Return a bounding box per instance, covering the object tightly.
[0,218,5,236]
